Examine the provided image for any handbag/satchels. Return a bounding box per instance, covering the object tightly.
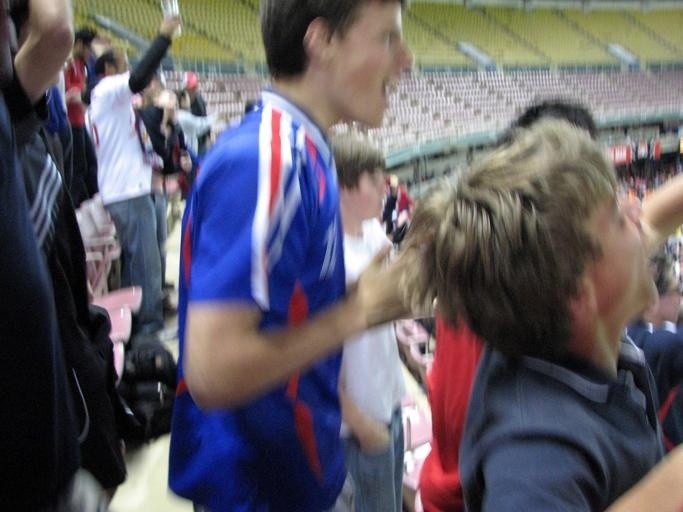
[122,344,176,440]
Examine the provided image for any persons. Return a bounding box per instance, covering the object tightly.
[0,0,682,511]
